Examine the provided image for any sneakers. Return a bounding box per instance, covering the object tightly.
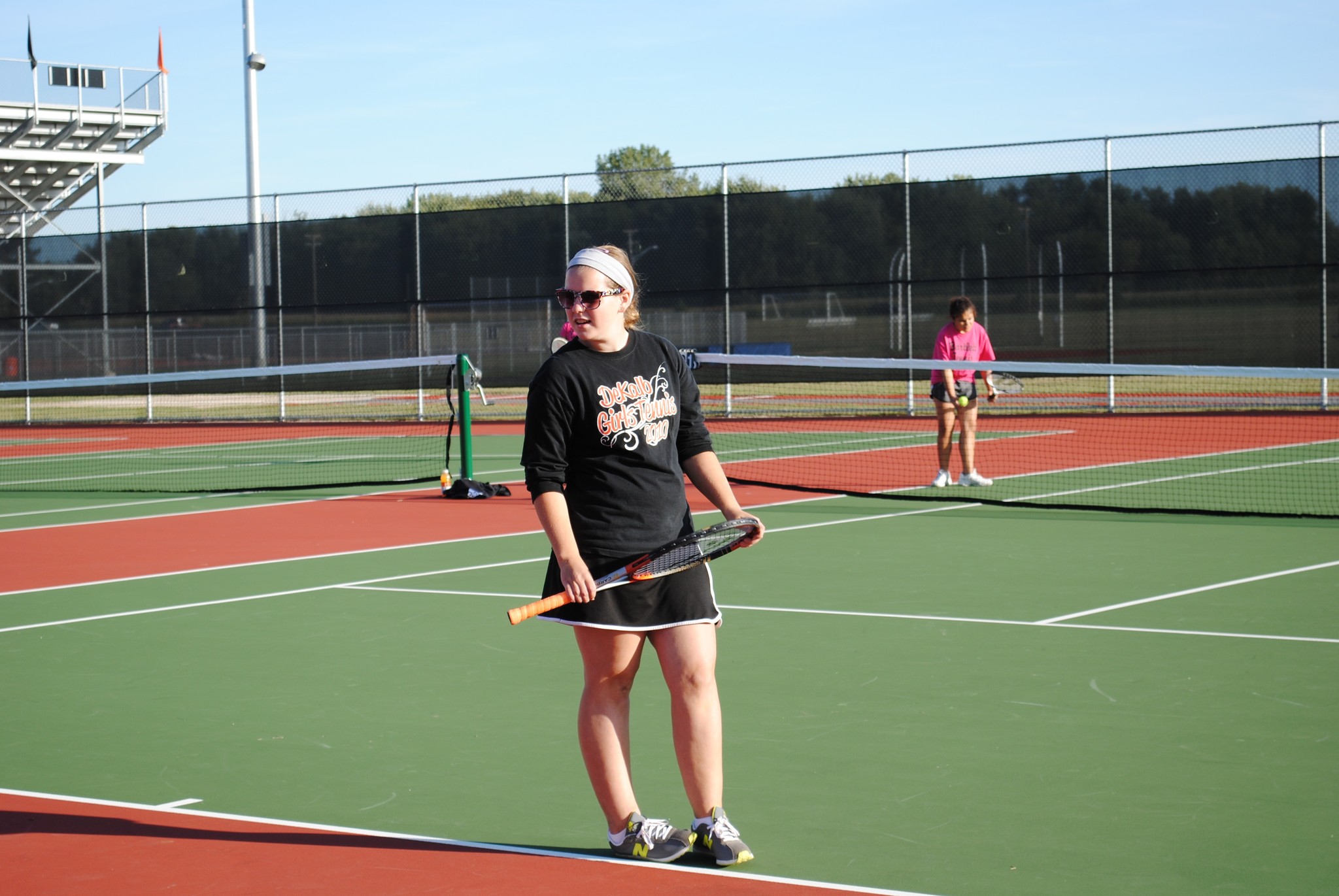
[609,812,697,863]
[691,806,754,866]
[932,469,953,487]
[959,468,993,487]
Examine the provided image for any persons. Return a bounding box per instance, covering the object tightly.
[520,245,765,866]
[928,296,996,488]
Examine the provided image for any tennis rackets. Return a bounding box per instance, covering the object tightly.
[986,371,1025,399]
[505,516,762,628]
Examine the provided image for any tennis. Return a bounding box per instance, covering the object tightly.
[959,396,969,407]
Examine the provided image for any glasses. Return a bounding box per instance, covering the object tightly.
[555,286,624,309]
